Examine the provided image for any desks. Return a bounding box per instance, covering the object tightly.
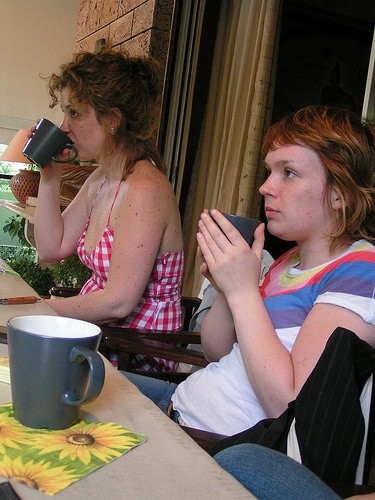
[0,200,67,273]
[0,259,259,500]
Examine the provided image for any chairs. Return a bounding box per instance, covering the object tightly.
[52,249,284,453]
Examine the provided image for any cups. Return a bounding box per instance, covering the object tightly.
[7,315,106,430]
[207,209,263,252]
[22,118,78,169]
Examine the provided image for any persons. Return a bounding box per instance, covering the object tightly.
[113,108,375,463]
[210,441,375,499]
[23,46,186,373]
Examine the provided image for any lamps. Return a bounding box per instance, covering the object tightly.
[0,129,40,205]
